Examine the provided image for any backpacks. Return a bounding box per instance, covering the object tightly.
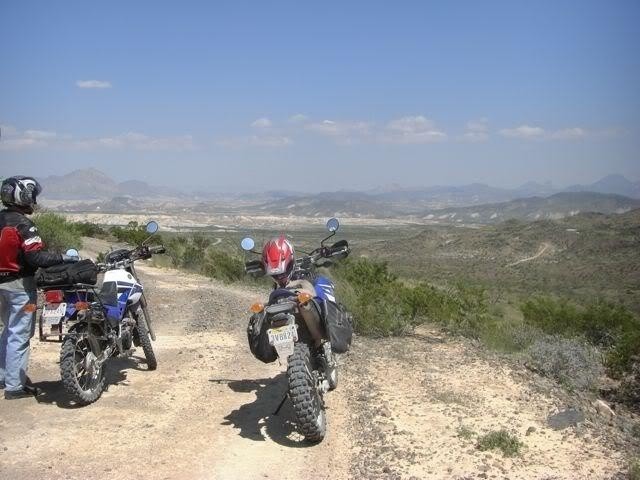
[38,258,97,287]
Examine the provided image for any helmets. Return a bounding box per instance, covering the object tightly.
[262,237,295,276]
[0,174,43,215]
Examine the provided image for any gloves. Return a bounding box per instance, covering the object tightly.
[62,254,95,265]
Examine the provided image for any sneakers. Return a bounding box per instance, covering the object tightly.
[0,376,42,400]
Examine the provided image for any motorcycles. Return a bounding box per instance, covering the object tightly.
[23,221,166,407]
[240,217,355,443]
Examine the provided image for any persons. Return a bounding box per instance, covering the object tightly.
[0,176,79,400]
